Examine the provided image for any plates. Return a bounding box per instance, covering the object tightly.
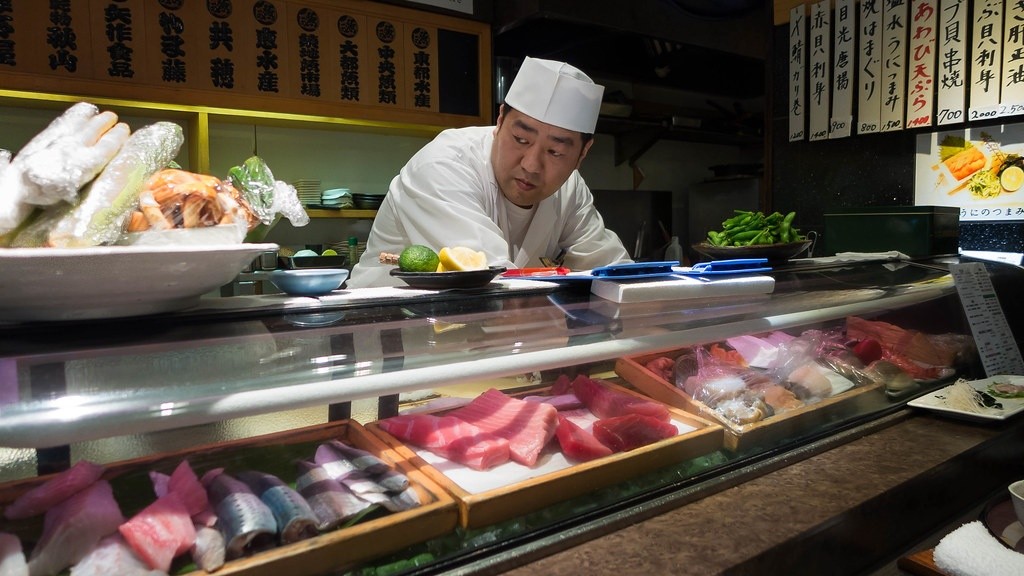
[690,240,813,262]
[294,250,318,256]
[322,249,337,256]
[397,300,503,322]
[907,374,1024,420]
[386,267,508,288]
[322,187,353,209]
[331,241,367,265]
[293,179,321,206]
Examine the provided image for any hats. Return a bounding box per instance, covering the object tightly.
[504,56,605,134]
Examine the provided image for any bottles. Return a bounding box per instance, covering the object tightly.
[348,237,358,268]
[664,235,683,262]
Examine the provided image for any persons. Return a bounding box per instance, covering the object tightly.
[344,57,635,290]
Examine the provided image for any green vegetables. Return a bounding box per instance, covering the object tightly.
[939,136,973,160]
[968,172,1000,198]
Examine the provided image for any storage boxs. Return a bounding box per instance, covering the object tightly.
[823,206,960,257]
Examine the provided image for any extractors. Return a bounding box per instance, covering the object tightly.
[590,77,760,159]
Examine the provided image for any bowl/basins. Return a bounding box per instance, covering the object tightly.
[286,313,346,329]
[0,242,279,322]
[352,192,386,210]
[1008,480,1024,525]
[268,269,349,297]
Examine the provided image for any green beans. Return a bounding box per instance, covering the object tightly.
[708,210,800,248]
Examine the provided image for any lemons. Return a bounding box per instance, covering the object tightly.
[1000,166,1024,192]
[439,247,486,271]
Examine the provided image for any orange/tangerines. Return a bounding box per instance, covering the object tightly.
[399,245,439,272]
